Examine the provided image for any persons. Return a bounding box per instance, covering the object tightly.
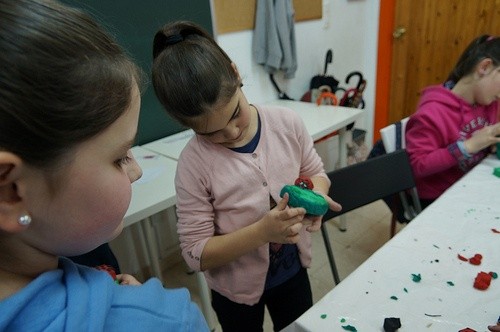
[406,33,500,212]
[150,18,343,332]
[1,0,213,332]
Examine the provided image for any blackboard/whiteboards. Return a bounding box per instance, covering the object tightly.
[1,0,220,151]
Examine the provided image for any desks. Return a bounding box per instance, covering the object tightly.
[120,95,500,332]
